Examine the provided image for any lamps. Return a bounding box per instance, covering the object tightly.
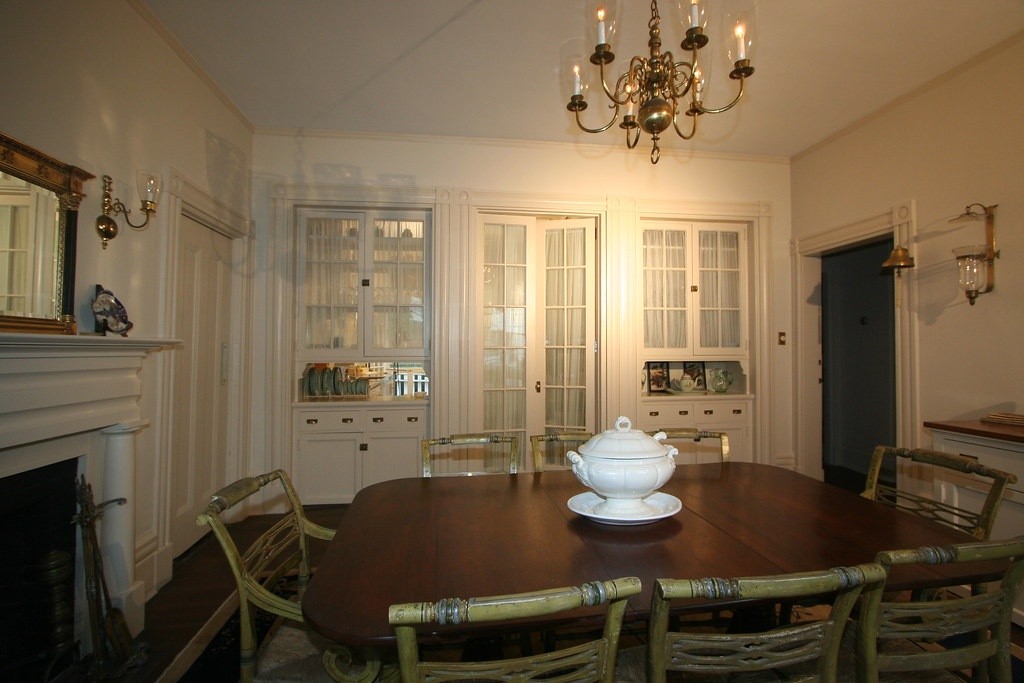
[564,0,756,165]
[946,202,999,305]
[96,170,161,250]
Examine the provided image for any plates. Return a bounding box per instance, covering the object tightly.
[566,492,683,525]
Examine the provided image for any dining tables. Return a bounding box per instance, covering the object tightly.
[303,462,990,683]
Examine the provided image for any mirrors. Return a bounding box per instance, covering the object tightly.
[2,130,93,337]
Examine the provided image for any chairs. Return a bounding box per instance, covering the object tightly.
[861,445,1019,543]
[421,429,729,478]
[195,468,415,683]
[388,577,642,683]
[615,536,1024,683]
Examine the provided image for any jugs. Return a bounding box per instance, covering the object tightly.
[712,368,733,393]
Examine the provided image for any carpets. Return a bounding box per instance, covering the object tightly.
[253,591,971,683]
[156,560,472,683]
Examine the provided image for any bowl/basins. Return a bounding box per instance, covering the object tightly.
[304,367,370,396]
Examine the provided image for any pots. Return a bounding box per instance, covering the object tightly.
[566,415,679,517]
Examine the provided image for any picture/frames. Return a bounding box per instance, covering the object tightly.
[684,362,706,390]
[648,361,670,392]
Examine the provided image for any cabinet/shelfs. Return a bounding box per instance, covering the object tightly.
[925,421,1024,629]
[292,201,430,504]
[642,392,755,464]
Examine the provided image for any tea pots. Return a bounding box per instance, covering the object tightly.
[675,373,699,393]
